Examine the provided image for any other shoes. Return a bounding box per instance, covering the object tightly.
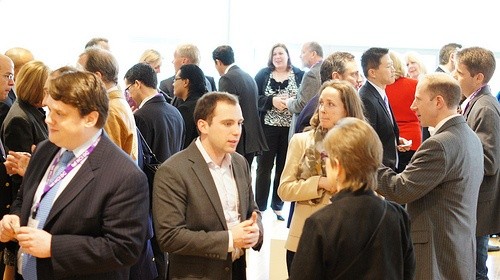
[272,208,285,221]
[487,244,500,251]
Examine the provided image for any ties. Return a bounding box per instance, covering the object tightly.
[22,150,75,280]
[384,95,394,124]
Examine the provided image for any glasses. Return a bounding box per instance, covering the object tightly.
[0,74,14,80]
[172,77,187,81]
[125,82,134,92]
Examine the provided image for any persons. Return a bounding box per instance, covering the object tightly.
[0,38,217,280]
[212,45,269,169]
[278,42,500,280]
[152,91,263,280]
[252,44,305,221]
